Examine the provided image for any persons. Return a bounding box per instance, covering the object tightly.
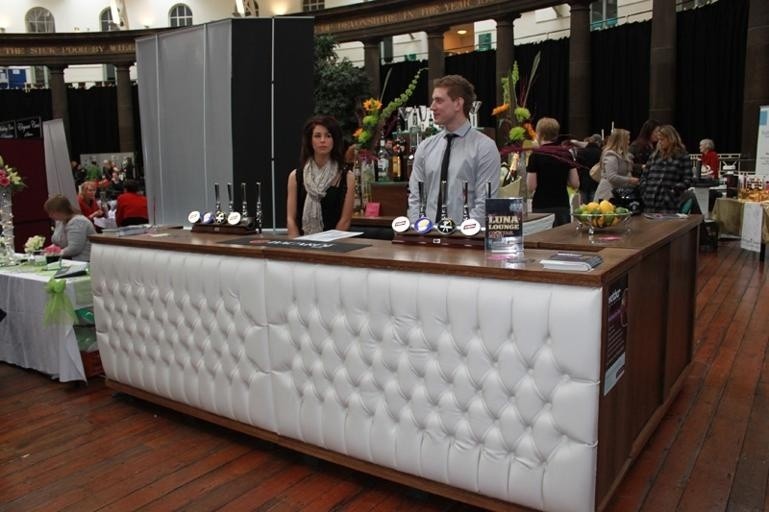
[77,182,107,221]
[287,114,355,243]
[526,117,719,216]
[43,193,96,261]
[407,74,501,233]
[116,180,149,227]
[71,157,133,198]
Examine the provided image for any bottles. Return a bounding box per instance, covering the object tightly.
[413,104,417,125]
[392,144,401,182]
[429,112,433,127]
[126,158,133,179]
[88,161,96,181]
[378,134,389,178]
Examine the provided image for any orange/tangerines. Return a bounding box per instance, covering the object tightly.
[615,207,629,218]
[605,211,616,223]
[574,208,602,221]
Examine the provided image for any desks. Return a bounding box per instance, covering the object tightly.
[0,253,94,385]
[709,198,769,262]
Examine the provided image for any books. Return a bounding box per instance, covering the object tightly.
[484,197,524,250]
[538,250,602,271]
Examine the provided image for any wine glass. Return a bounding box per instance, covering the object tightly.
[468,101,484,130]
[419,105,427,132]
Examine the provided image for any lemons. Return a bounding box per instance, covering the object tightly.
[611,217,622,226]
[591,216,604,227]
[585,201,600,212]
[598,200,614,215]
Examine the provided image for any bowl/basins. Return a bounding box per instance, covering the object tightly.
[569,211,632,229]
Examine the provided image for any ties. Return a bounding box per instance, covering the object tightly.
[434,133,457,226]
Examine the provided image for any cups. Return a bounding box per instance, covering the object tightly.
[727,176,738,187]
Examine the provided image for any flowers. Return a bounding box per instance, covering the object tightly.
[354,67,430,161]
[0,155,28,192]
[490,50,542,146]
